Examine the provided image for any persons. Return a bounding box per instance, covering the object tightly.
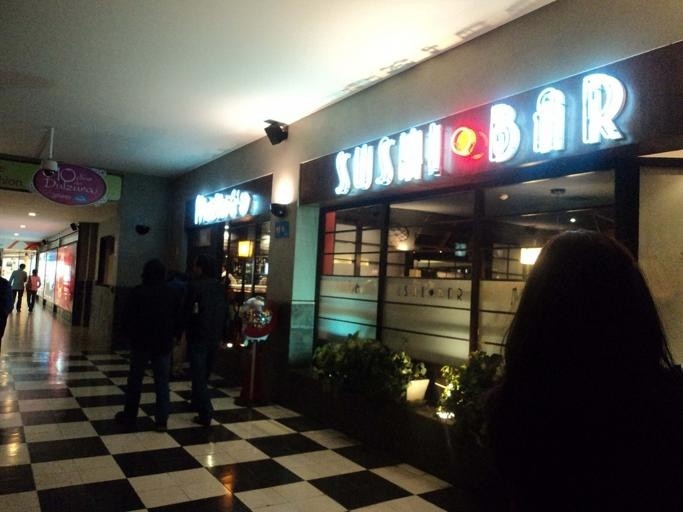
[8,264,41,312]
[0,277,11,344]
[449,228,683,512]
[114,246,227,432]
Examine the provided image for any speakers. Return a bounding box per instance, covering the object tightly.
[264,124,283,146]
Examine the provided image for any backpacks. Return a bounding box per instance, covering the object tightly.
[26,276,32,292]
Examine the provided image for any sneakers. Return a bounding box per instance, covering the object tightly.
[114,412,133,432]
[192,415,211,426]
[151,423,167,431]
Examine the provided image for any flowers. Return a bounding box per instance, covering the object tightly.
[300,333,427,389]
[434,348,502,420]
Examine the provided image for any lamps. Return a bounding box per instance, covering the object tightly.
[263,124,289,145]
[270,204,288,218]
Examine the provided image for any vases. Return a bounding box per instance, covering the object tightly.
[397,374,431,403]
[432,379,448,402]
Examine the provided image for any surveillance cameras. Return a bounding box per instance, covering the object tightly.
[40,159,58,177]
